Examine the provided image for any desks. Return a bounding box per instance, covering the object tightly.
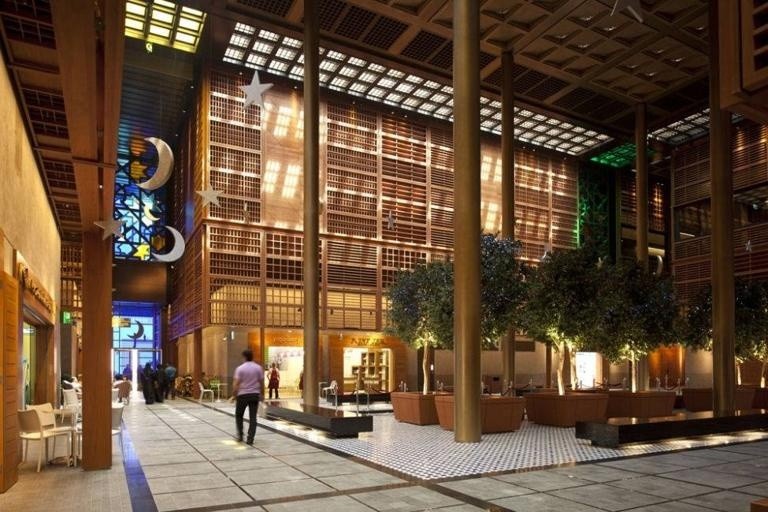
[210,383,228,402]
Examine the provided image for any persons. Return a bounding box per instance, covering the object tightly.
[298,365,304,398]
[231,349,264,446]
[138,361,176,405]
[123,364,132,381]
[112,374,123,387]
[266,362,281,400]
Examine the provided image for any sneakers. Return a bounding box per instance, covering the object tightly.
[144,394,176,405]
[236,434,243,442]
[246,436,254,445]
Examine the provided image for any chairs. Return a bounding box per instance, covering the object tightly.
[198,382,214,402]
[321,380,338,400]
[175,375,192,397]
[18,380,131,472]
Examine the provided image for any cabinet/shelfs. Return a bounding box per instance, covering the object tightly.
[361,349,394,393]
